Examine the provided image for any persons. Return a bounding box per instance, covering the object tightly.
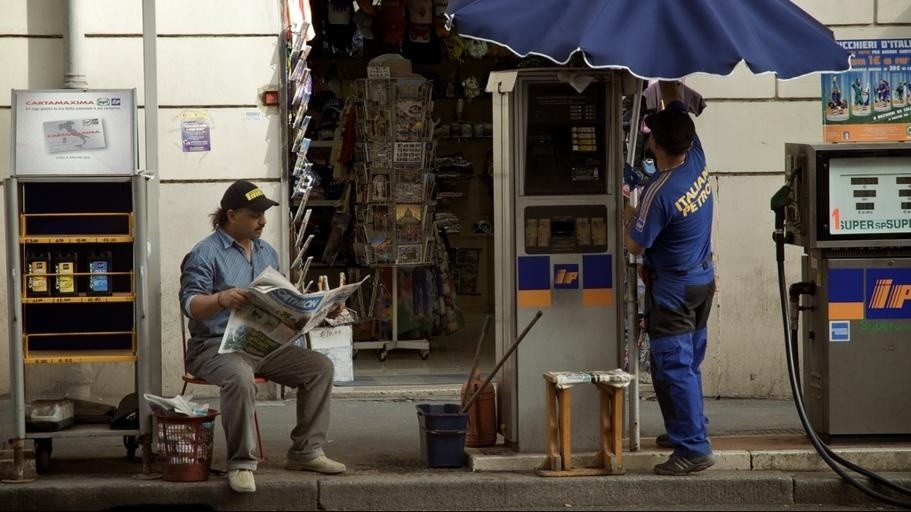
[624,102,715,477]
[372,174,388,200]
[178,180,347,493]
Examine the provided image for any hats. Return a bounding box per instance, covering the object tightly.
[222,181,280,213]
[646,111,696,149]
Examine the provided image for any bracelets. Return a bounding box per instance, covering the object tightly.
[218,292,225,308]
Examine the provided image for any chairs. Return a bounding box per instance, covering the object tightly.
[176,287,270,461]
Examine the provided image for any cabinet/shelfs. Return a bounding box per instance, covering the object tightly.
[3,174,154,479]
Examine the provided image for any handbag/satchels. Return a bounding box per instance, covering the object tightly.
[322,210,353,268]
[354,236,466,340]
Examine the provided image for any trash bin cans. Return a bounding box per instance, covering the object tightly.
[152,409,221,482]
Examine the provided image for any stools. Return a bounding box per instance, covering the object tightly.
[540,368,631,477]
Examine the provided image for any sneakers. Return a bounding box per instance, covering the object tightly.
[655,432,677,449]
[653,451,715,476]
[228,469,257,494]
[283,452,347,474]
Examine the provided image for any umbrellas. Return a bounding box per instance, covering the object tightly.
[443,0,855,452]
